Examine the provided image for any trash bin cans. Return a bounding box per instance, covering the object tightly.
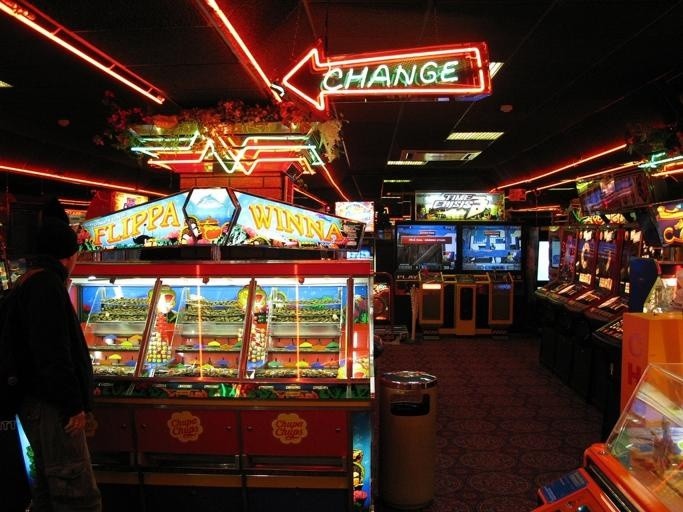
[377,371,440,510]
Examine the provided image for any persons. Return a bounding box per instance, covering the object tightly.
[8,202,107,511]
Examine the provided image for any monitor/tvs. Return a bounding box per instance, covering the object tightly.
[460,221,527,274]
[547,226,658,298]
[395,220,460,274]
